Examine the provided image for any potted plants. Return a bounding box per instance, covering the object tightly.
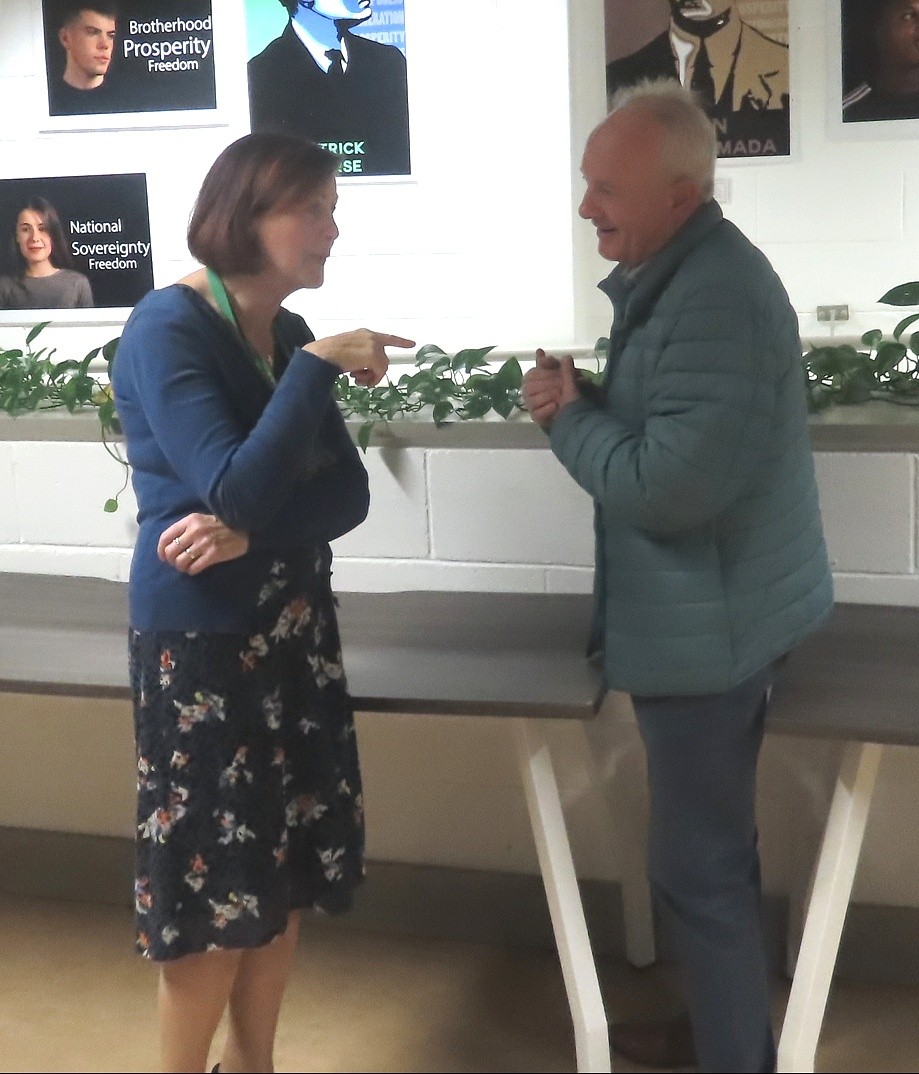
[0,280,919,574]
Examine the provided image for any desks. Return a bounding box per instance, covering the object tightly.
[0,570,612,1073]
[763,602,919,1073]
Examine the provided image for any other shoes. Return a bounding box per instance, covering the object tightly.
[610,1006,695,1066]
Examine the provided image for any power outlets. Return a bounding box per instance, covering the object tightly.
[817,305,849,320]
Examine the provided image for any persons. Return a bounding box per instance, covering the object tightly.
[0,196,95,309]
[112,134,417,1073]
[842,0,919,120]
[523,81,835,1073]
[51,0,157,114]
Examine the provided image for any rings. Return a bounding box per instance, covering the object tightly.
[174,539,188,552]
[185,548,197,561]
